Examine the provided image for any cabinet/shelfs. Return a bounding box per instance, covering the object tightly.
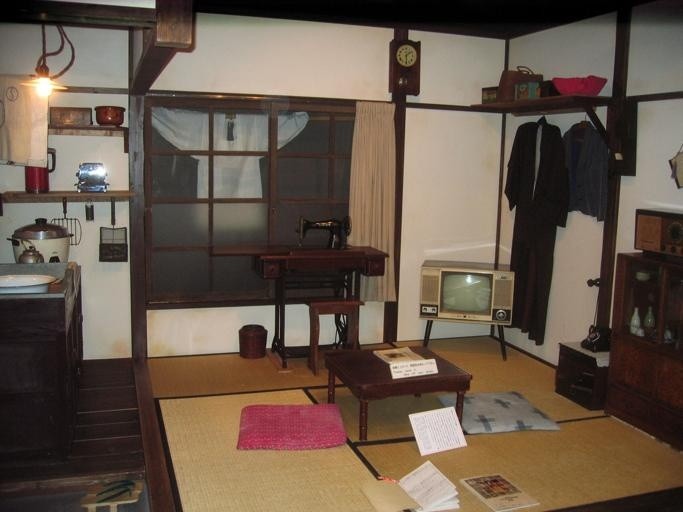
[628,306,675,347]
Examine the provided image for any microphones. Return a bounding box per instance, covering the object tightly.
[304,300,363,375]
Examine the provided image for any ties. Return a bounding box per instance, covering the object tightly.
[419,260,515,326]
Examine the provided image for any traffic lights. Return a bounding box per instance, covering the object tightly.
[431,390,562,435]
[236,403,345,452]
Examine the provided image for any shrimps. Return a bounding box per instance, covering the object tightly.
[6,217,74,262]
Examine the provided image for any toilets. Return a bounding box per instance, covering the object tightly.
[582,325,612,352]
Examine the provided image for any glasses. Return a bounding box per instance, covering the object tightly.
[20,22,69,102]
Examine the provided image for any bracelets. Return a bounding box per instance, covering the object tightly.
[0,274,58,295]
[92,104,126,127]
[550,74,607,97]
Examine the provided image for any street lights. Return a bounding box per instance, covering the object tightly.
[498,69,544,101]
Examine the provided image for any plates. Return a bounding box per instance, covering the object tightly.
[0,124,132,226]
[553,341,609,413]
[1,287,83,463]
[605,251,682,447]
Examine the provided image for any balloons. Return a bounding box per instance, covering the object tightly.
[388,39,421,95]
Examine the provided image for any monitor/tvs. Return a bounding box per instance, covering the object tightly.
[372,346,428,365]
[460,474,538,512]
[356,461,460,512]
[406,406,469,456]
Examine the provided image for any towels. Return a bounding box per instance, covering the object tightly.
[239,324,267,359]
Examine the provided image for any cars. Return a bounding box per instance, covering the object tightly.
[636,272,650,282]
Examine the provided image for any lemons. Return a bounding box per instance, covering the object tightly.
[17,245,44,264]
